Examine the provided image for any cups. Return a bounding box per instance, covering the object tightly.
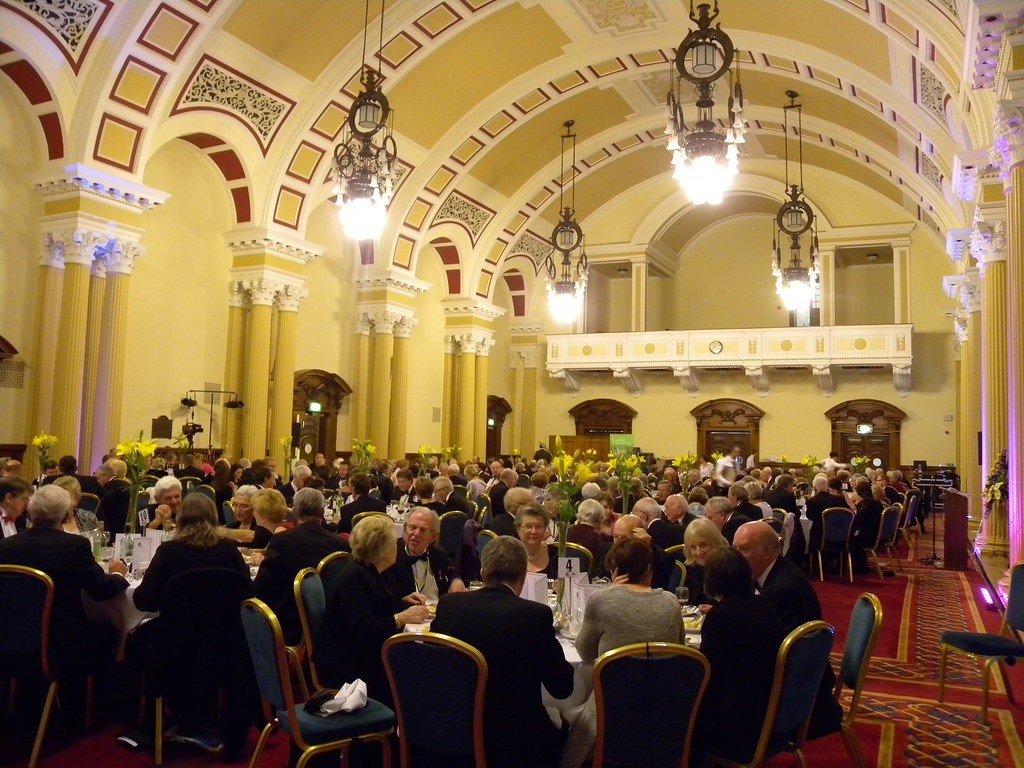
[469,581,484,591]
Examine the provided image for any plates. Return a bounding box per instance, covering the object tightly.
[681,611,702,617]
[682,623,701,633]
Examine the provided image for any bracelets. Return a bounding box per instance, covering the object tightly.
[394,613,401,629]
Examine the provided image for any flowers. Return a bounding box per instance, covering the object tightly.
[32,431,870,609]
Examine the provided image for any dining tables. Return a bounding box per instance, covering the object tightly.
[73,546,261,660]
[399,584,703,712]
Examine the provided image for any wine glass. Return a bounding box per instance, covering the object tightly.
[386,500,411,523]
[675,587,689,614]
[547,578,555,605]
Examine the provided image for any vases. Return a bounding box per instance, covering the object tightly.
[123,483,141,557]
[550,519,570,631]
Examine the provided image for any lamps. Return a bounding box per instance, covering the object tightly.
[770,88,824,313]
[618,268,627,274]
[332,1,398,245]
[866,254,878,260]
[665,0,746,205]
[546,120,589,323]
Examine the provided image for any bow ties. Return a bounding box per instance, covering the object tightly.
[0,511,15,524]
[408,552,427,565]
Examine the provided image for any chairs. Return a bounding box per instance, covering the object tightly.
[938,560,1024,723]
[0,479,931,768]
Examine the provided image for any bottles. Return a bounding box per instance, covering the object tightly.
[93,520,177,579]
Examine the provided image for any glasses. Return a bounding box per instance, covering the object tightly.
[232,503,253,510]
[518,524,545,530]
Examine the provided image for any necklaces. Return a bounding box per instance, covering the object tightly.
[528,544,543,560]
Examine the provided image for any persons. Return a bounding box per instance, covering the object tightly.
[0,442,912,768]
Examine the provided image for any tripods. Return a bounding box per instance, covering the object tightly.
[173,435,195,455]
